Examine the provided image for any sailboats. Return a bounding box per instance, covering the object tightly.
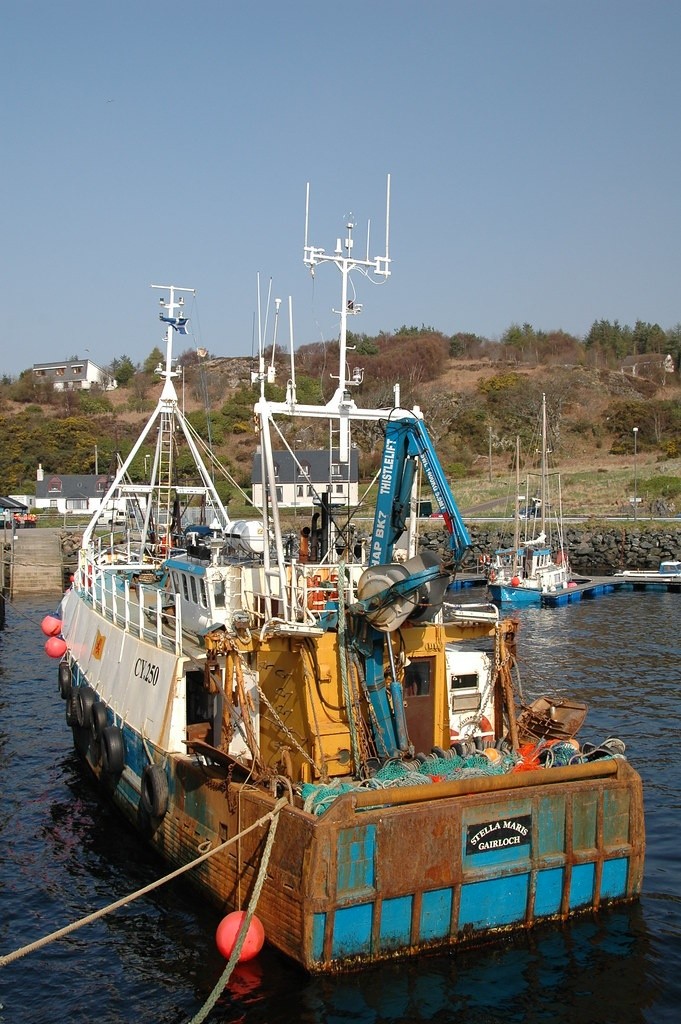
[488,393,618,607]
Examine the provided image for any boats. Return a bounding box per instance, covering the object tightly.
[43,171,645,970]
[613,562,681,588]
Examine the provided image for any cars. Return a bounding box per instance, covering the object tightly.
[513,507,541,517]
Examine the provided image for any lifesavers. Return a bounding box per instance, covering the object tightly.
[450,712,495,751]
[57,661,72,700]
[103,725,126,775]
[63,687,109,750]
[141,763,169,818]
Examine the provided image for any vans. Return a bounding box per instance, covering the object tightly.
[116,510,127,525]
